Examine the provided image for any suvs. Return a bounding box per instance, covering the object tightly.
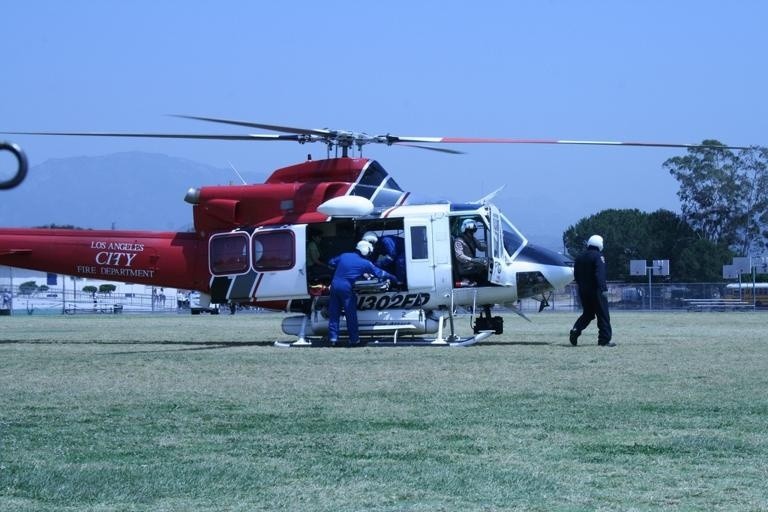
[187,288,220,315]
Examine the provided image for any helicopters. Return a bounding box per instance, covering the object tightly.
[0,110,758,349]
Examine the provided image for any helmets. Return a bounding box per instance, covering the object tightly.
[356,241,373,255]
[587,234,604,252]
[362,231,378,243]
[460,219,477,233]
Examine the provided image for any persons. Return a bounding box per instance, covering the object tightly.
[327,240,398,347]
[153,286,194,309]
[569,234,618,348]
[228,303,236,315]
[2,288,10,309]
[306,228,336,286]
[362,230,405,291]
[453,218,494,275]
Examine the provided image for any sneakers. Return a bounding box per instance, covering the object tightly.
[349,341,364,347]
[570,328,578,346]
[329,339,339,346]
[599,341,617,347]
